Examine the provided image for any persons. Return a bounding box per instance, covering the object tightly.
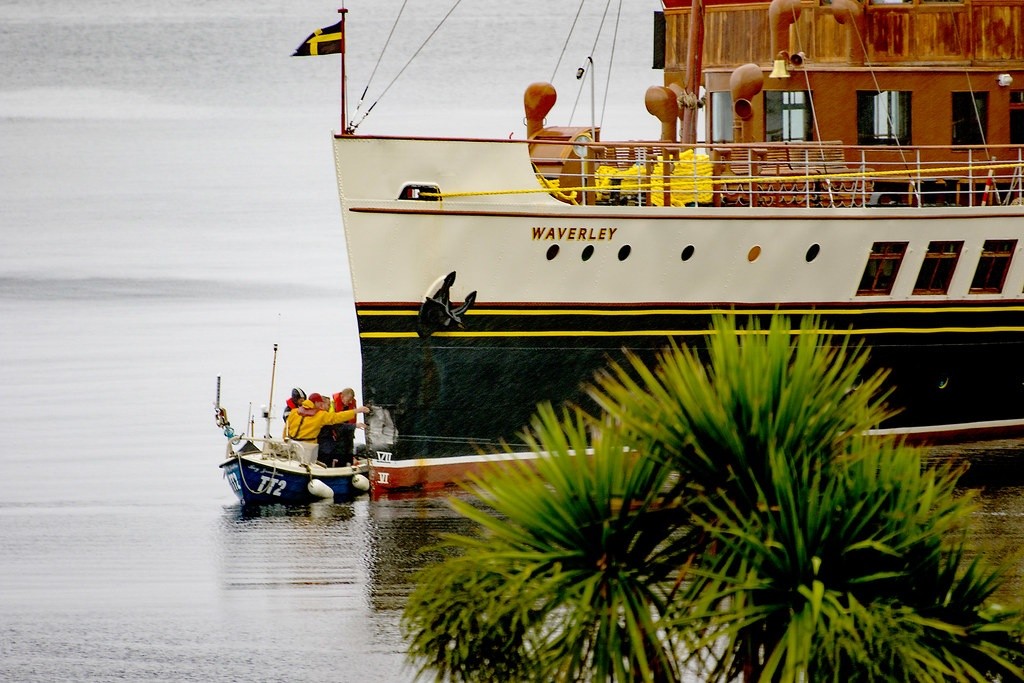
[332,388,357,464]
[283,393,370,460]
[318,395,365,461]
[283,386,307,423]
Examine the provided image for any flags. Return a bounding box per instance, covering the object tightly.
[288,19,345,58]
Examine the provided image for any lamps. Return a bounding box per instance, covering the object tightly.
[768,50,804,82]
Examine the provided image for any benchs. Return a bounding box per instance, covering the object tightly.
[726,139,876,208]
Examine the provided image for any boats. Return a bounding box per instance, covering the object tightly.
[212,343,370,518]
[333,0,1023,504]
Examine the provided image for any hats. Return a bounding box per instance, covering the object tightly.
[309,393,325,403]
